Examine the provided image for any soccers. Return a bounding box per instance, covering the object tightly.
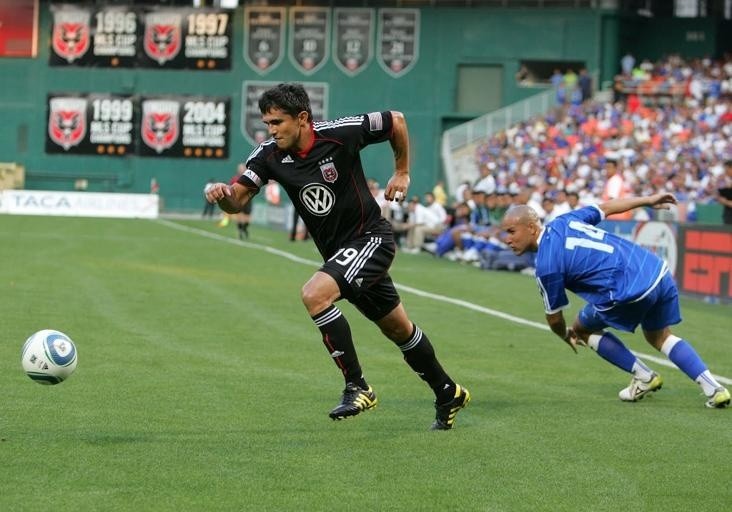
[21,329,78,385]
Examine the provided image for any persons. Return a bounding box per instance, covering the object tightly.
[368,177,450,256]
[229,163,252,239]
[502,191,731,409]
[421,56,731,275]
[202,83,471,430]
[203,177,216,216]
[286,200,309,241]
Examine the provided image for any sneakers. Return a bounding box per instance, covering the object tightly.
[618,371,663,402]
[431,383,471,431]
[704,388,731,409]
[330,382,378,421]
